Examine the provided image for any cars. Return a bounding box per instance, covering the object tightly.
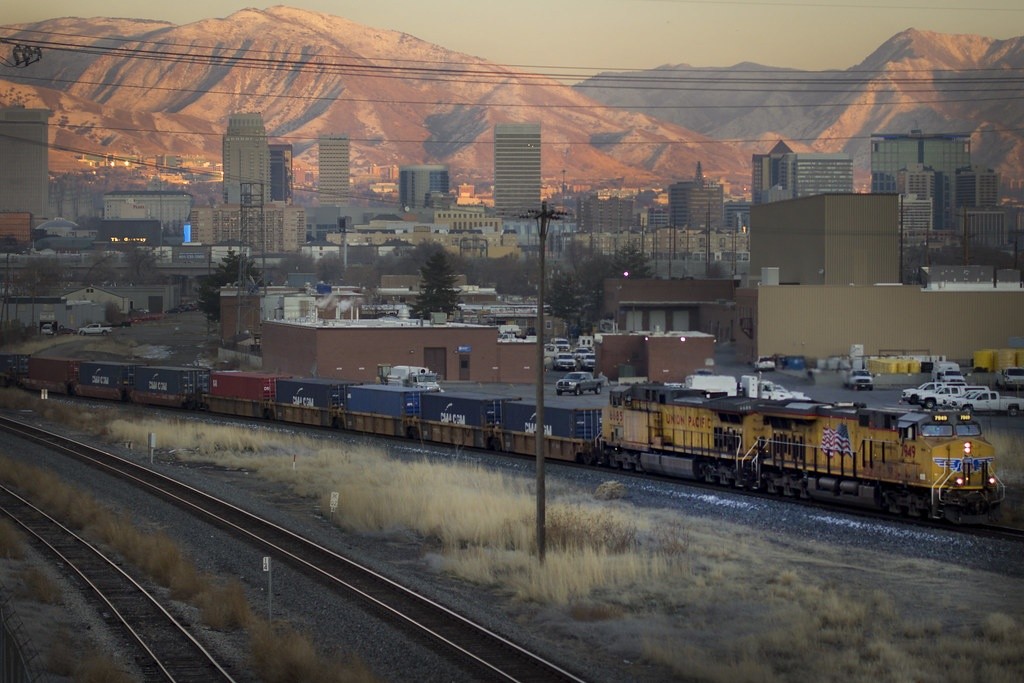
[164,308,184,314]
[42,324,53,335]
[552,352,576,371]
[580,353,596,371]
[844,370,875,390]
[996,367,1024,389]
[572,348,593,360]
[551,338,570,351]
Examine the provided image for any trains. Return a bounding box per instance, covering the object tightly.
[0,354,1006,524]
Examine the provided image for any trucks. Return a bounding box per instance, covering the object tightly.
[390,365,440,393]
[932,361,967,382]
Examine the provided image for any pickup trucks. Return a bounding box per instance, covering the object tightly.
[901,382,967,404]
[941,390,1024,417]
[555,372,604,396]
[917,386,989,409]
[755,357,776,372]
[498,334,522,342]
[78,324,113,336]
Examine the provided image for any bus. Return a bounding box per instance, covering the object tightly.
[179,253,204,259]
